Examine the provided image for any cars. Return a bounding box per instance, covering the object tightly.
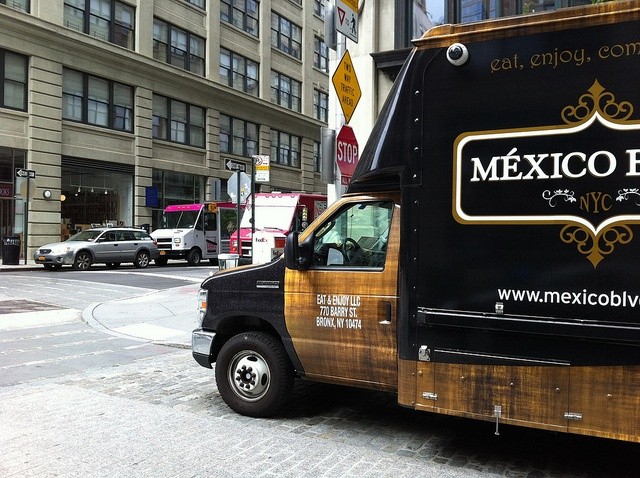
[33,226,158,270]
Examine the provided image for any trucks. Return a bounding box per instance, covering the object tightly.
[149,201,246,265]
[229,191,327,267]
[191,0,640,444]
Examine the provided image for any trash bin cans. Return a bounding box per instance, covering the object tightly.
[2,235,21,265]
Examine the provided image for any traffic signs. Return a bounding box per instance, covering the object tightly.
[224,157,247,173]
[13,166,39,189]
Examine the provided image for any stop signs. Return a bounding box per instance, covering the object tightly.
[336,125,359,176]
